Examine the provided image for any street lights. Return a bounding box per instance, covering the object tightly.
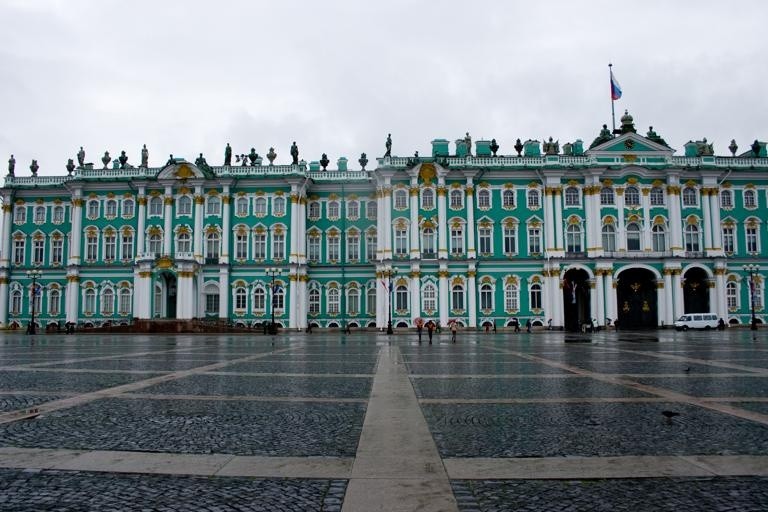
[381,266,398,334]
[27,270,43,334]
[742,264,760,330]
[265,267,282,323]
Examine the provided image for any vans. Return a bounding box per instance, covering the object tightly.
[675,313,718,331]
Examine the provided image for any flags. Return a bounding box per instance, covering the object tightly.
[610,71,622,100]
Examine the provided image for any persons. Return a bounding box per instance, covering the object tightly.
[464,131,472,153]
[426,320,435,344]
[717,318,725,330]
[306,322,312,333]
[25,321,31,334]
[345,323,351,334]
[486,316,619,333]
[449,321,457,343]
[417,320,424,341]
[646,126,656,138]
[70,325,74,334]
[65,323,69,334]
[385,133,392,154]
[8,141,299,174]
[600,124,611,138]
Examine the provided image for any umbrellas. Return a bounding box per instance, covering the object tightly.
[447,317,457,325]
[65,321,70,326]
[70,322,76,325]
[413,317,425,326]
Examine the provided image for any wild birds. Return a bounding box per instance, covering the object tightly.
[684,367,690,372]
[661,411,680,418]
